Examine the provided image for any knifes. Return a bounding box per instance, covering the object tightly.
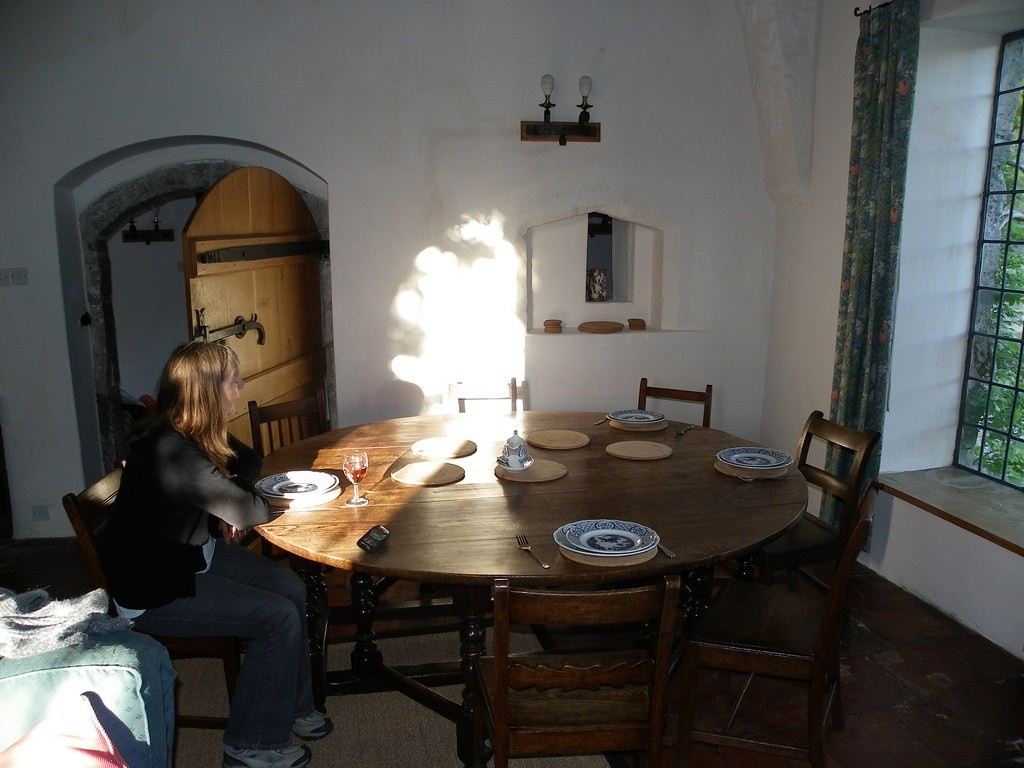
[270,508,339,513]
[593,416,608,426]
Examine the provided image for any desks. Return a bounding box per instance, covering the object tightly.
[247,410,809,768]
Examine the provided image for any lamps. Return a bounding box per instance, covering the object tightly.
[122,216,174,245]
[520,74,601,145]
[734,0,822,202]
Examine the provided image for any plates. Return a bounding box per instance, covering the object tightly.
[606,409,667,425]
[553,519,660,557]
[566,521,656,554]
[721,448,787,467]
[254,470,341,500]
[716,447,794,470]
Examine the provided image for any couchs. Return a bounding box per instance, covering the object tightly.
[0,587,178,768]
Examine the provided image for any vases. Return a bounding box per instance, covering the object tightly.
[587,268,608,302]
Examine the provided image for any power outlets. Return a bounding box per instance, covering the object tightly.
[32,504,50,521]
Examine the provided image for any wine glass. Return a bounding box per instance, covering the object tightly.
[342,450,369,507]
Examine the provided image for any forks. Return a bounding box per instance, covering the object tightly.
[515,533,550,569]
[675,425,695,435]
[733,471,793,483]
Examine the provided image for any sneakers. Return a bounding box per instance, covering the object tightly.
[291,710,333,742]
[223,740,311,768]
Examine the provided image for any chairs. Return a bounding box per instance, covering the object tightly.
[448,376,881,768]
[247,387,330,458]
[126,394,157,441]
[63,458,245,731]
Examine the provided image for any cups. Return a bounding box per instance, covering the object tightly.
[586,268,607,302]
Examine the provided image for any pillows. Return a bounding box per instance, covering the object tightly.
[0,695,129,768]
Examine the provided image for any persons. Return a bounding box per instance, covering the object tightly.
[104,339,333,768]
